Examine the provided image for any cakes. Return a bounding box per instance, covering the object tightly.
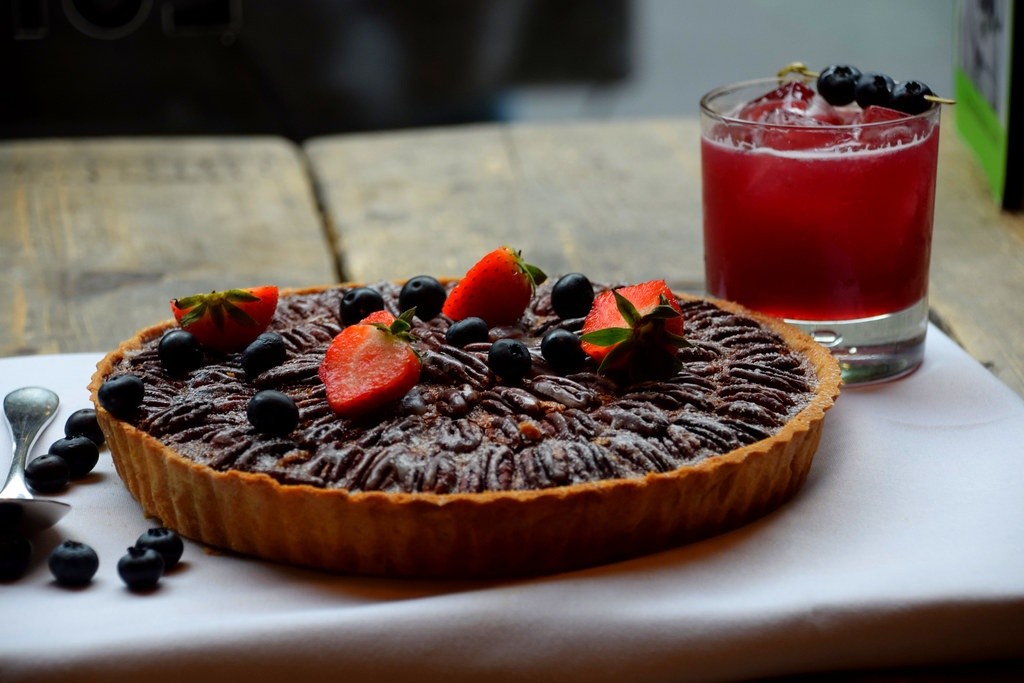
[86,244,843,576]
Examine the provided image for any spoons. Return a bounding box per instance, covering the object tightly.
[0,386,72,530]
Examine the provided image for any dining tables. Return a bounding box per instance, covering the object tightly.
[1,111,1024,683]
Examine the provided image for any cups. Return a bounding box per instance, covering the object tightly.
[699,79,943,390]
